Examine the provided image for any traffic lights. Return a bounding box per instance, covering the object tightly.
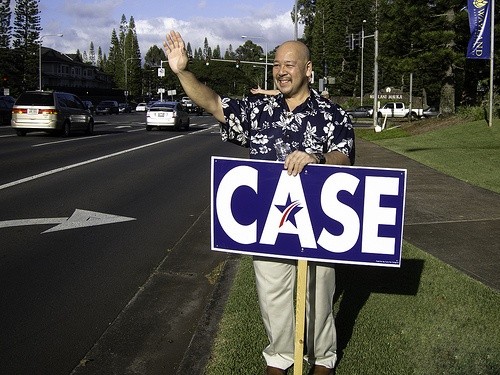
[346,35,354,50]
[356,32,365,48]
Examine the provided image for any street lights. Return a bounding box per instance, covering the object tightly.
[125,58,143,103]
[361,20,366,105]
[241,35,268,96]
[39,34,63,90]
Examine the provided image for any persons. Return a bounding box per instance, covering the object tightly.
[162,29,356,375]
[250,85,281,96]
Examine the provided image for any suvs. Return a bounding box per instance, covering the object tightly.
[146,102,190,132]
[96,100,119,115]
[11,91,94,137]
[182,97,203,116]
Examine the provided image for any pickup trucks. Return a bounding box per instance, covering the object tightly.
[366,102,423,121]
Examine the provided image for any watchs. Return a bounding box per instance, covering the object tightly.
[313,153,327,165]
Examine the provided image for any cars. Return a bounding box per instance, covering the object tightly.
[345,106,373,119]
[119,103,131,113]
[81,101,95,116]
[0,96,16,126]
[423,107,439,118]
[136,103,146,112]
[146,101,157,112]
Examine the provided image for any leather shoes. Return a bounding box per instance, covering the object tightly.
[313,365,331,375]
[266,366,284,375]
